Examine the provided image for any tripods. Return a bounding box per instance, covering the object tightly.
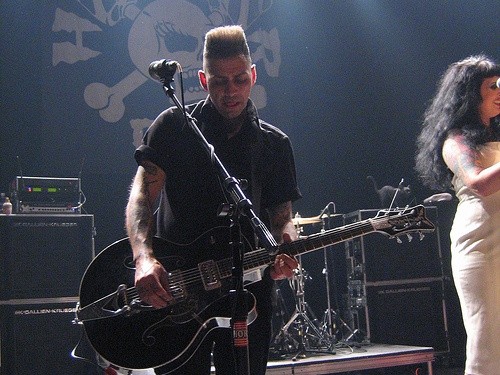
[269,225,372,359]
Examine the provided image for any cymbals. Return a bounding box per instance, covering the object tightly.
[319,213,344,219]
[291,218,323,225]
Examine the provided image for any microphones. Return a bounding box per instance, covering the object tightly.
[318,203,331,219]
[149,58,178,81]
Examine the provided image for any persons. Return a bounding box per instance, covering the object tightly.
[124,26,302,375]
[414,56,500,375]
[348,174,424,309]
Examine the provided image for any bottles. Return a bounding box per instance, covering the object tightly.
[0,193,12,214]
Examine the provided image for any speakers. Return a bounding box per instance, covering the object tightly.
[1,304,104,374]
[346,205,445,286]
[0,214,97,302]
[358,281,450,357]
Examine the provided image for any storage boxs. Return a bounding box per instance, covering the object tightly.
[342,205,450,356]
[0,214,100,375]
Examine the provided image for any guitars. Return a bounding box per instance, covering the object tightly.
[76,205,436,375]
